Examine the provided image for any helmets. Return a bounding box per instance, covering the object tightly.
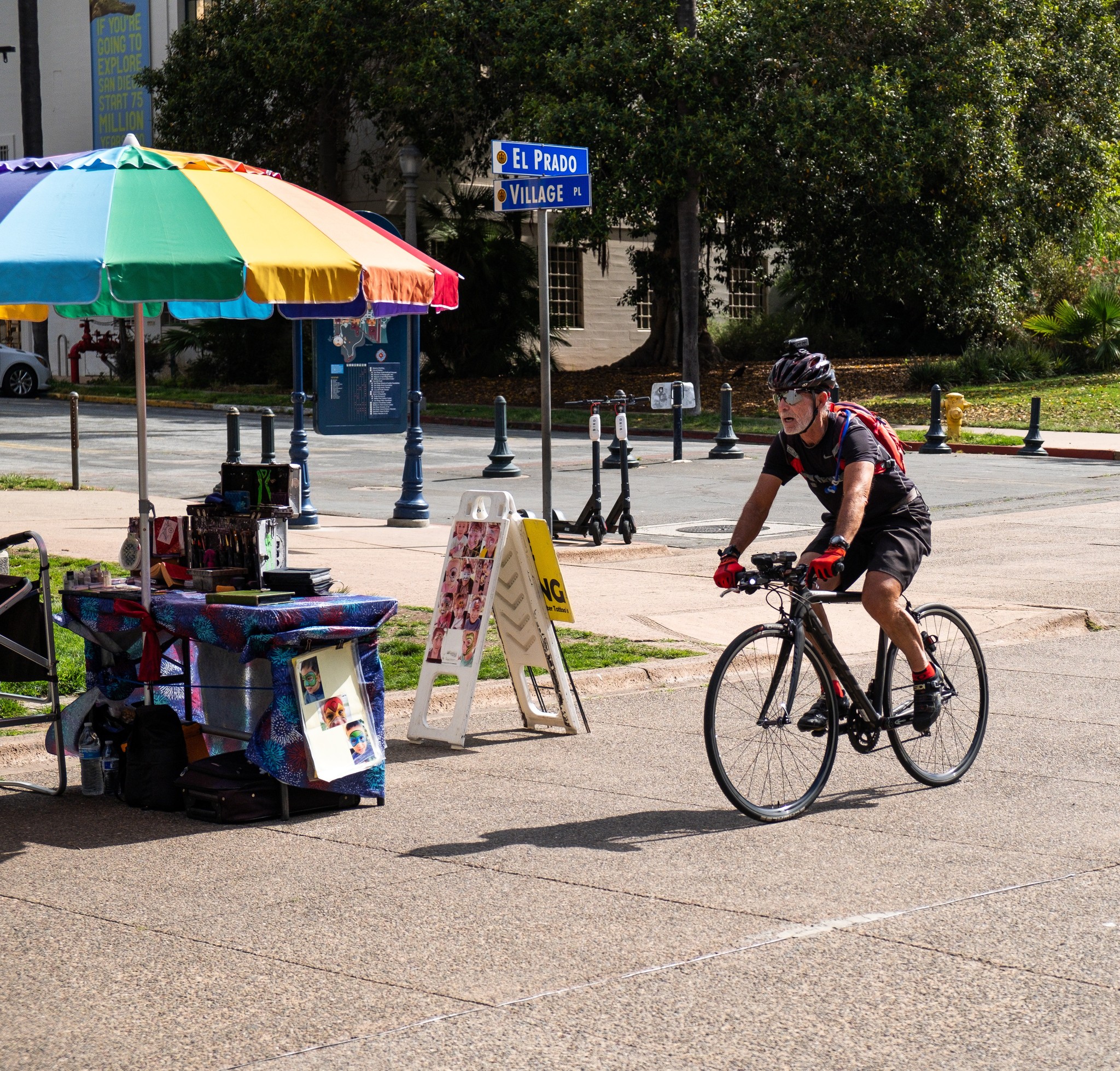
[765,349,838,393]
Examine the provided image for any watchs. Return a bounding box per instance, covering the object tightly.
[717,545,740,560]
[829,535,850,551]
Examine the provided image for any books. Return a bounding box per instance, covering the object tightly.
[204,589,296,607]
[140,562,193,587]
[263,568,334,595]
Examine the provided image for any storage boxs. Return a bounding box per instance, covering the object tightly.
[191,515,277,588]
[221,462,302,518]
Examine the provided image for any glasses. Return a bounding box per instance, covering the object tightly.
[773,388,815,406]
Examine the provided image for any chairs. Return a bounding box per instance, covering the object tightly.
[0,531,74,796]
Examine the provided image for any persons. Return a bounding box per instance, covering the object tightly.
[426,521,500,668]
[319,697,347,728]
[300,656,325,705]
[713,350,943,733]
[345,719,376,764]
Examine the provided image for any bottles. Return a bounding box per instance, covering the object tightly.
[97,567,103,582]
[90,568,98,583]
[78,723,104,795]
[84,571,91,584]
[103,571,112,586]
[78,574,84,585]
[66,571,74,587]
[101,741,121,796]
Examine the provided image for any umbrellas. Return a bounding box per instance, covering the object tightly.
[0,131,459,708]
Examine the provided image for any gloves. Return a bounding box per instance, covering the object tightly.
[713,554,746,588]
[805,544,845,590]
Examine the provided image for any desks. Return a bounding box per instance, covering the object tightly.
[56,578,398,820]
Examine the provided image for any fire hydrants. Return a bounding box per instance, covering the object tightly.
[940,392,975,439]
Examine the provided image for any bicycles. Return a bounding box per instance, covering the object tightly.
[702,551,990,823]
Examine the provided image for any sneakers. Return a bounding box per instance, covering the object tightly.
[913,661,944,733]
[798,688,850,731]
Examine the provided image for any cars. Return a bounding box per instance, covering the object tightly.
[0,343,54,399]
